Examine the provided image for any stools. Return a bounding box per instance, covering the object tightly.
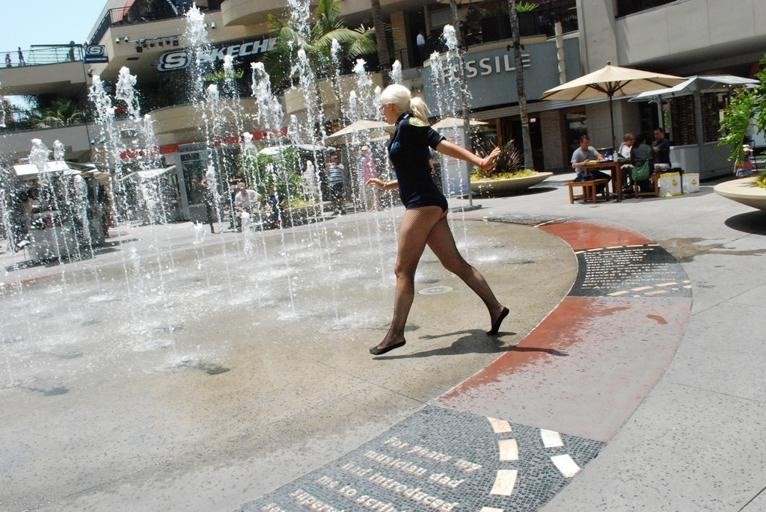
[568,172,660,204]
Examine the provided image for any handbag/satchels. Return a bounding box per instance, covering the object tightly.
[617,153,630,164]
[631,161,650,181]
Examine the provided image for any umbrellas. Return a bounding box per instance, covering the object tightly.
[431,117,489,129]
[319,118,396,146]
[538,61,689,193]
[257,143,329,157]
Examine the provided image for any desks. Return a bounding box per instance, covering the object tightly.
[584,160,630,201]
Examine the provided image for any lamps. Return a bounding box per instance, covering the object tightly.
[204,21,215,30]
[116,35,128,43]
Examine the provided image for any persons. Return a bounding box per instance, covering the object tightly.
[324,151,348,216]
[18,186,109,241]
[190,176,288,232]
[618,128,672,196]
[18,47,25,64]
[360,146,379,210]
[365,83,510,356]
[570,134,612,198]
[5,54,12,68]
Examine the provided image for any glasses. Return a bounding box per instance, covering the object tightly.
[378,103,395,115]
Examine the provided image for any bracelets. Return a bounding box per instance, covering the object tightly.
[382,181,388,190]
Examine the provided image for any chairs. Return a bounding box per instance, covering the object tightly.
[744,139,758,172]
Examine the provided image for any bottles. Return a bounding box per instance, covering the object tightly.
[613,150,619,163]
